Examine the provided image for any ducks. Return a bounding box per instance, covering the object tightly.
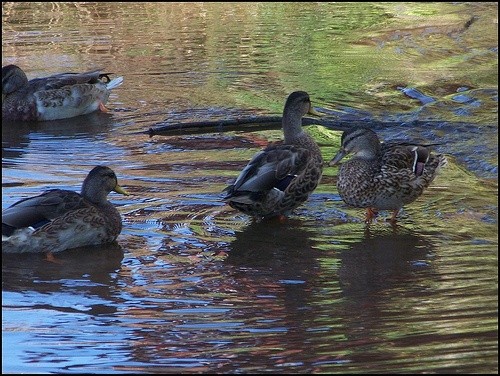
[1,64,123,123]
[2,165,131,255]
[219,91,327,224]
[328,126,447,226]
